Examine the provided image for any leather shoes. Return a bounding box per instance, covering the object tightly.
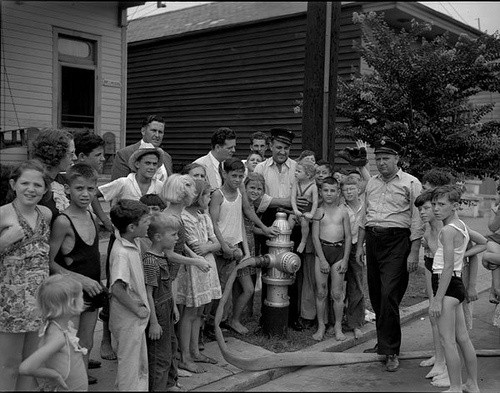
[386,354,398,372]
[364,344,378,353]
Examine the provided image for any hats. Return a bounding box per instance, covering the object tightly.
[128,142,164,171]
[271,128,295,144]
[374,139,401,154]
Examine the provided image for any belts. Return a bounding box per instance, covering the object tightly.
[365,225,411,233]
[433,269,461,278]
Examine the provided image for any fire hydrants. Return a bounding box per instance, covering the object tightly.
[261,212,301,335]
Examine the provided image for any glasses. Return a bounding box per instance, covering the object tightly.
[66,150,76,158]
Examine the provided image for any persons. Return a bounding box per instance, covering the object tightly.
[109,198,151,393]
[19,273,89,393]
[0,158,53,393]
[249,131,266,160]
[355,140,422,372]
[293,140,370,342]
[5,130,77,217]
[245,150,263,175]
[191,128,236,193]
[139,192,167,215]
[50,164,109,384]
[111,114,173,181]
[182,180,222,372]
[253,127,304,331]
[162,170,213,388]
[142,212,181,393]
[414,169,499,392]
[181,163,206,181]
[90,143,165,360]
[73,130,106,173]
[229,171,280,335]
[209,157,281,335]
